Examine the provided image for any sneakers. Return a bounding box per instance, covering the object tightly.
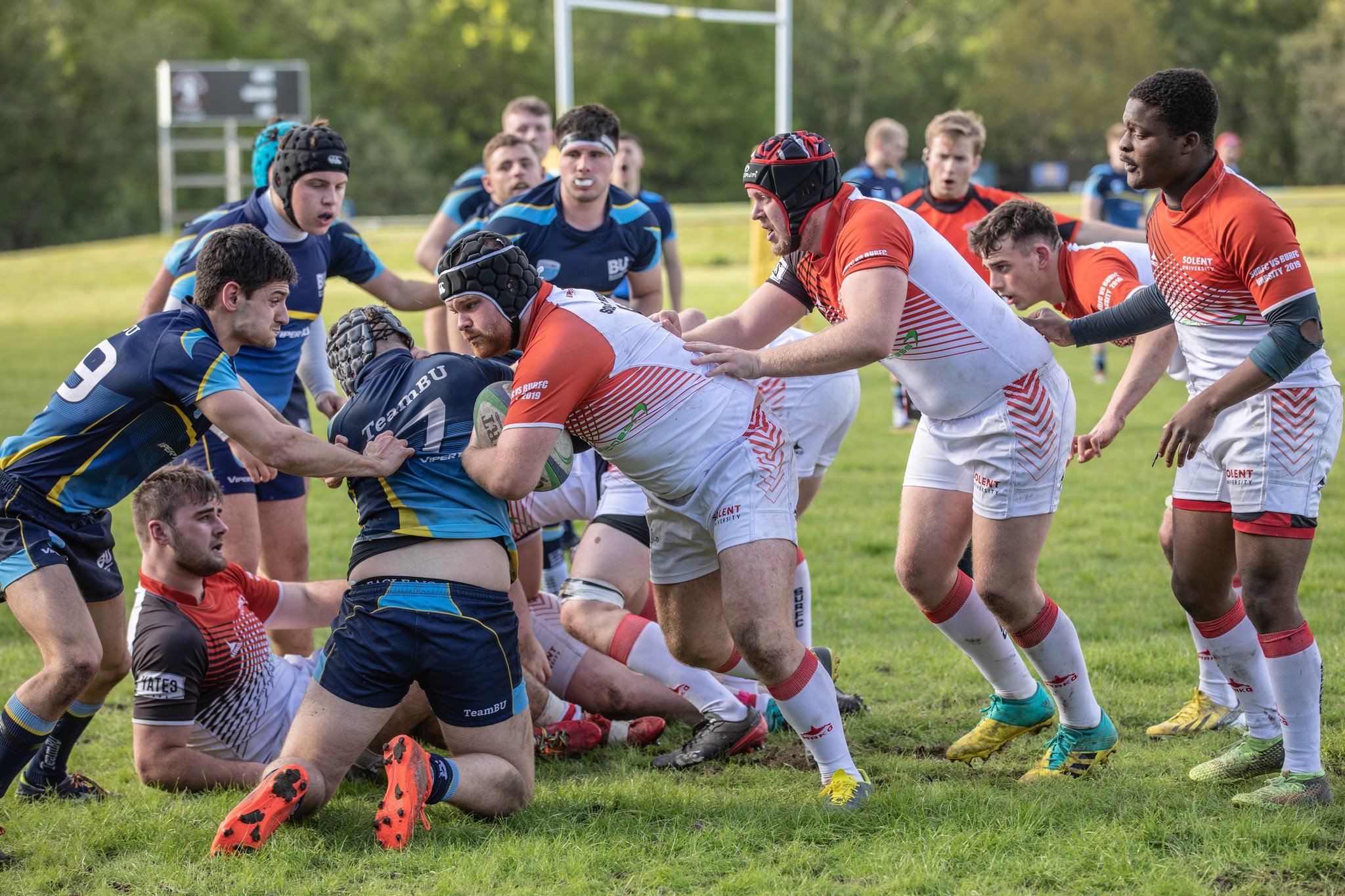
[945,680,1058,770]
[834,685,873,717]
[651,704,769,769]
[209,765,309,857]
[1147,687,1247,737]
[1017,708,1117,784]
[0,826,8,861]
[585,710,666,748]
[1189,735,1285,785]
[1232,770,1335,813]
[749,693,784,734]
[534,719,602,755]
[819,768,872,813]
[15,769,119,802]
[805,646,840,769]
[373,735,432,854]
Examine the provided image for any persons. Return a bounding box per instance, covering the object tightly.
[122,67,1342,813]
[0,224,416,804]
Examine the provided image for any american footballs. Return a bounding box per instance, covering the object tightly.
[473,381,573,493]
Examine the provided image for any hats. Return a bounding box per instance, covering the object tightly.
[252,120,303,189]
[324,307,414,398]
[270,125,351,232]
[435,230,542,322]
[742,130,843,253]
